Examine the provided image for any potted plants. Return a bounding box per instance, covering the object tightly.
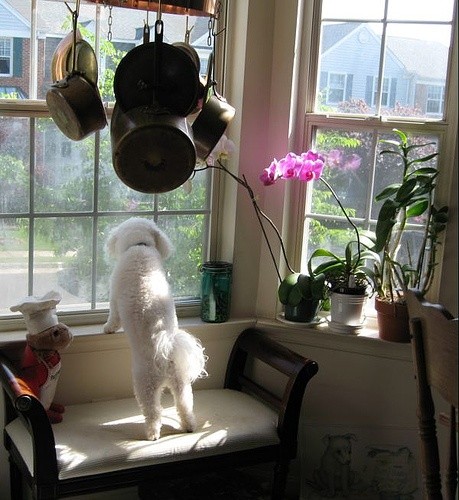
[368,128,442,344]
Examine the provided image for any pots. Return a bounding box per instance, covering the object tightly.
[50,26,99,86]
[111,19,201,193]
[46,10,107,142]
[189,32,236,161]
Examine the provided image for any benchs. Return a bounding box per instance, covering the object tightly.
[0,325,318,500]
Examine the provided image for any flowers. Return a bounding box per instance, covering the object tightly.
[260,147,377,311]
[183,134,299,281]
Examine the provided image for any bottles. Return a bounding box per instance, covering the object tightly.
[197,259,234,324]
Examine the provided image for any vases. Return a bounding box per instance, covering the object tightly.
[273,280,320,322]
[327,287,369,334]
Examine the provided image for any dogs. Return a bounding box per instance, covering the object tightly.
[104,216,209,442]
